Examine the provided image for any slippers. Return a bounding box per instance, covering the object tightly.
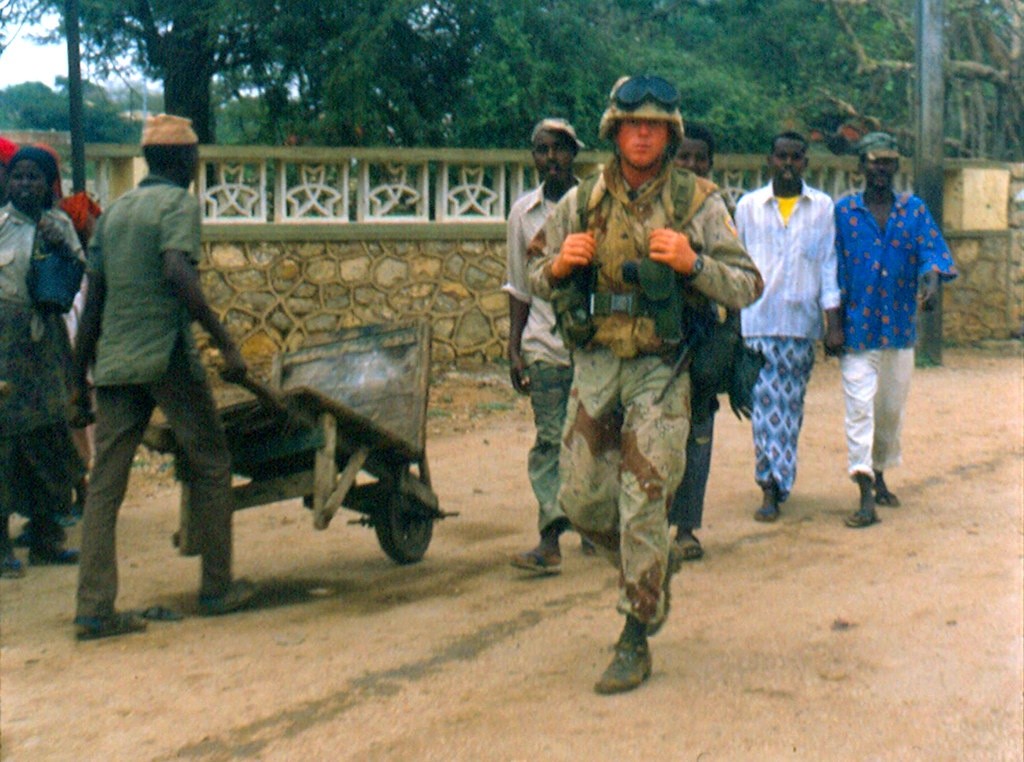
[509,553,563,573]
[581,539,595,553]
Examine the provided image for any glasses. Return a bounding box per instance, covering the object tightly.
[611,76,683,112]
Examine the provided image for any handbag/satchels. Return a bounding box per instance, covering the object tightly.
[30,250,85,313]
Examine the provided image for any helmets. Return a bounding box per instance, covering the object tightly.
[599,76,685,146]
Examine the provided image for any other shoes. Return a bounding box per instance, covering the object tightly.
[754,501,779,520]
[874,489,901,509]
[29,545,81,566]
[673,535,702,559]
[74,614,143,641]
[203,582,257,614]
[0,554,25,577]
[846,511,878,527]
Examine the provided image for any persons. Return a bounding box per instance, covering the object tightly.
[66,113,267,642]
[500,116,598,573]
[737,130,845,523]
[667,125,742,561]
[836,132,958,528]
[0,136,105,579]
[544,75,763,694]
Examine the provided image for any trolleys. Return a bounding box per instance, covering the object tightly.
[137,323,460,567]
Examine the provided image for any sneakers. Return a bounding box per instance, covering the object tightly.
[600,640,652,689]
[645,559,670,639]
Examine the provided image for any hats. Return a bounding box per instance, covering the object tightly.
[856,132,902,162]
[139,112,198,145]
[533,117,585,156]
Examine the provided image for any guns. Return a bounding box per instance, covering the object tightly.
[654,323,709,404]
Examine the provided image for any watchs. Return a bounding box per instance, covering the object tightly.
[691,253,703,280]
